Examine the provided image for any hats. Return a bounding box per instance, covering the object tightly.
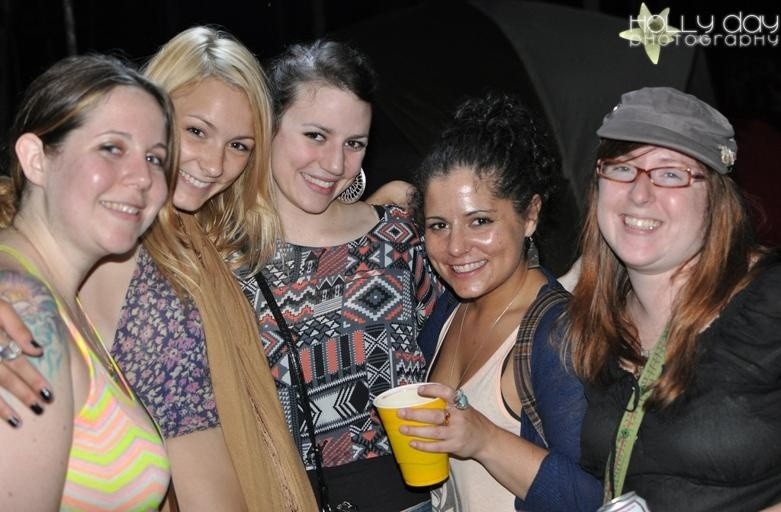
[595,86,739,175]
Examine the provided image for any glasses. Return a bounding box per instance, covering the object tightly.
[595,161,704,188]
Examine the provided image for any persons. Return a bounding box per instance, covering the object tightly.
[1,28,780,512]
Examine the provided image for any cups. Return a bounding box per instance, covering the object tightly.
[373,382,451,488]
